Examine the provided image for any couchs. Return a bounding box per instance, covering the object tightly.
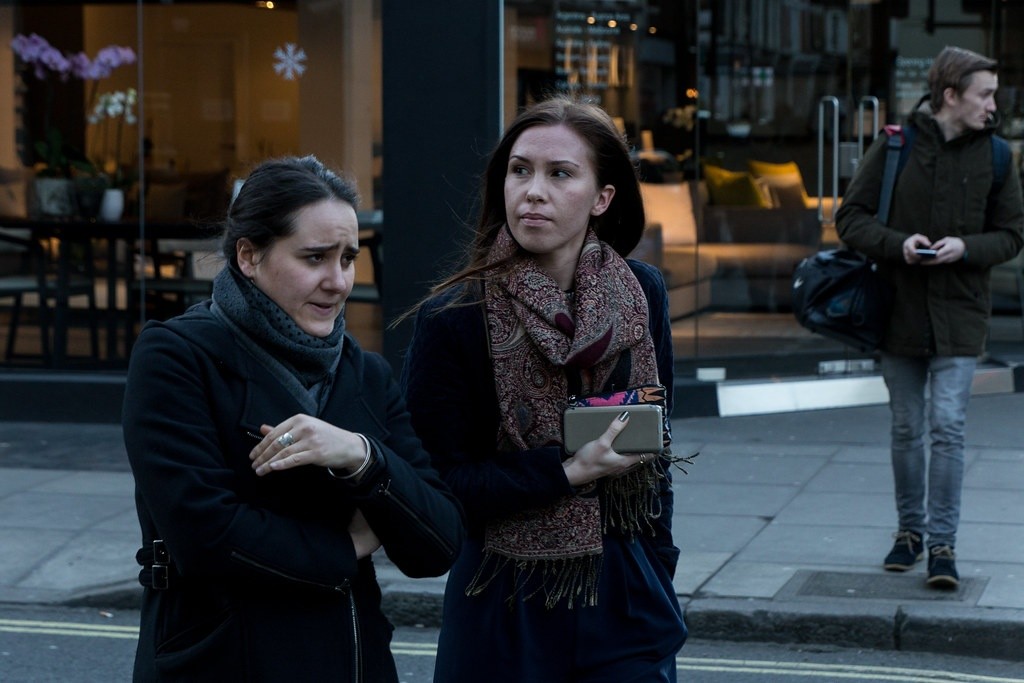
[627,181,843,320]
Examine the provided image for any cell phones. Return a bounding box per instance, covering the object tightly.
[562,405,663,456]
[916,248,936,258]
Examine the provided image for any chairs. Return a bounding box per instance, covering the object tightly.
[0,210,122,370]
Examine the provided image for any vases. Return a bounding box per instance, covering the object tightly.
[100,191,124,220]
[30,177,67,221]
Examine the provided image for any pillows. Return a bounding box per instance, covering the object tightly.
[703,167,767,212]
[744,159,809,209]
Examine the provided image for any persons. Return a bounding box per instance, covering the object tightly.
[397,98,689,683]
[120,151,461,683]
[833,45,1024,584]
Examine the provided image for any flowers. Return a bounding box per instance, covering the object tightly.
[8,34,139,181]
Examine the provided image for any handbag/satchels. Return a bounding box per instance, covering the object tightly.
[789,249,890,352]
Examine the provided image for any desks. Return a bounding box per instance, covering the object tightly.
[42,214,224,364]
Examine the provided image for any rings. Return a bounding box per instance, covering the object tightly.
[639,451,647,465]
[278,432,295,447]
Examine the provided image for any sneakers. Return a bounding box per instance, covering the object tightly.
[926,544,961,586]
[884,529,923,570]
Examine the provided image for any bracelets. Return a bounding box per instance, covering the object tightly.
[326,431,371,479]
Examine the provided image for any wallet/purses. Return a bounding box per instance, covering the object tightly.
[570,385,673,448]
